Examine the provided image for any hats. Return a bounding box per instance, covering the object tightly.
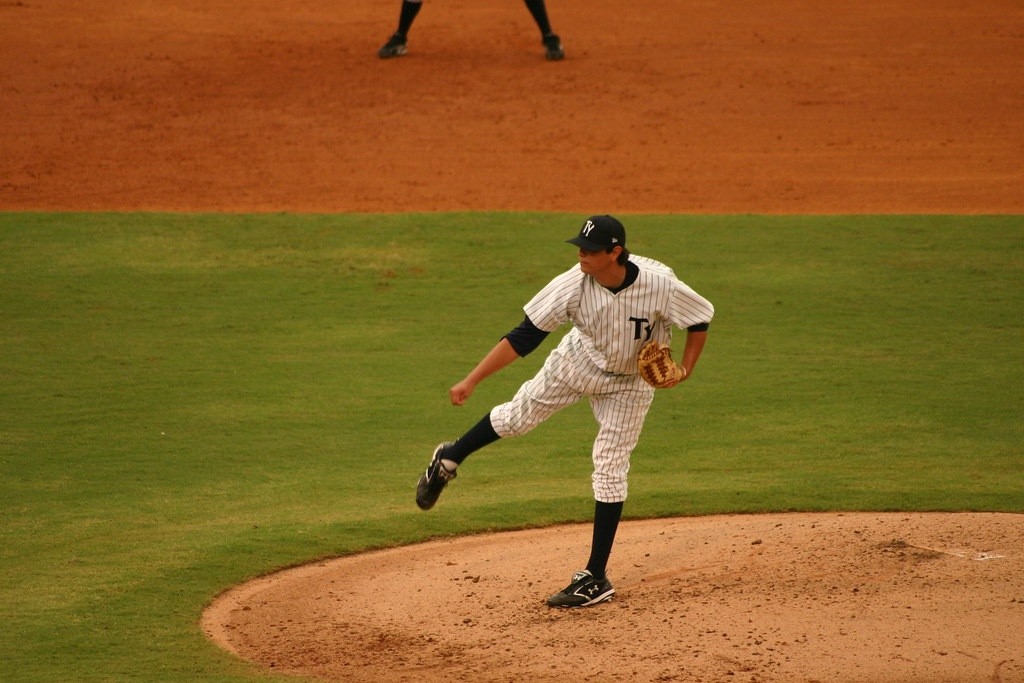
[566,214,627,252]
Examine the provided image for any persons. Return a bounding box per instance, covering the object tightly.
[417,215,715,609]
[377,0,565,60]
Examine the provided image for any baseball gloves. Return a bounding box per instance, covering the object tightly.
[635,340,687,390]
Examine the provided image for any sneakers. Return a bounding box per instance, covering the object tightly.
[542,30,564,61]
[415,441,458,510]
[547,570,616,608]
[379,32,408,58]
